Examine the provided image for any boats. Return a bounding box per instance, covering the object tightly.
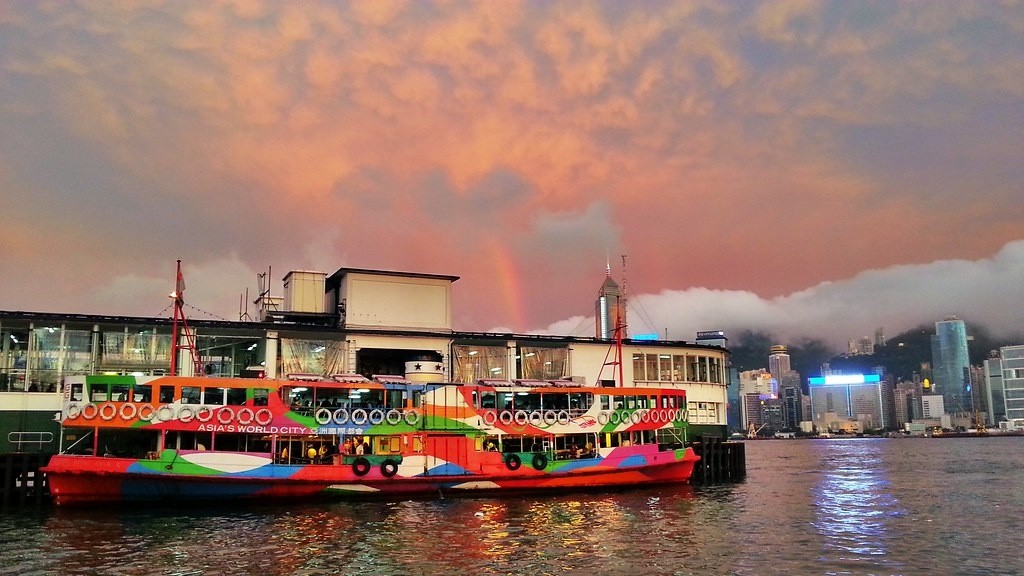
[35,253,706,510]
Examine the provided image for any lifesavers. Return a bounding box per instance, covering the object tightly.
[676,409,689,422]
[558,410,570,426]
[531,454,548,471]
[236,408,254,425]
[382,459,399,477]
[119,402,137,421]
[529,412,542,426]
[386,410,401,426]
[66,403,81,420]
[630,411,641,424]
[651,410,659,423]
[352,457,370,476]
[597,411,609,425]
[351,408,367,425]
[157,405,175,422]
[668,409,675,422]
[514,410,528,425]
[99,402,117,420]
[506,454,521,471]
[659,409,668,422]
[609,410,620,425]
[620,410,631,424]
[368,409,384,425]
[404,410,419,425]
[641,410,650,423]
[544,409,557,425]
[315,408,332,425]
[176,405,195,423]
[333,409,349,425]
[255,408,273,426]
[81,402,98,420]
[137,404,156,421]
[196,405,214,422]
[499,410,513,426]
[216,408,234,425]
[483,410,498,426]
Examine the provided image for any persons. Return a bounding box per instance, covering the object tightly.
[277,438,366,464]
